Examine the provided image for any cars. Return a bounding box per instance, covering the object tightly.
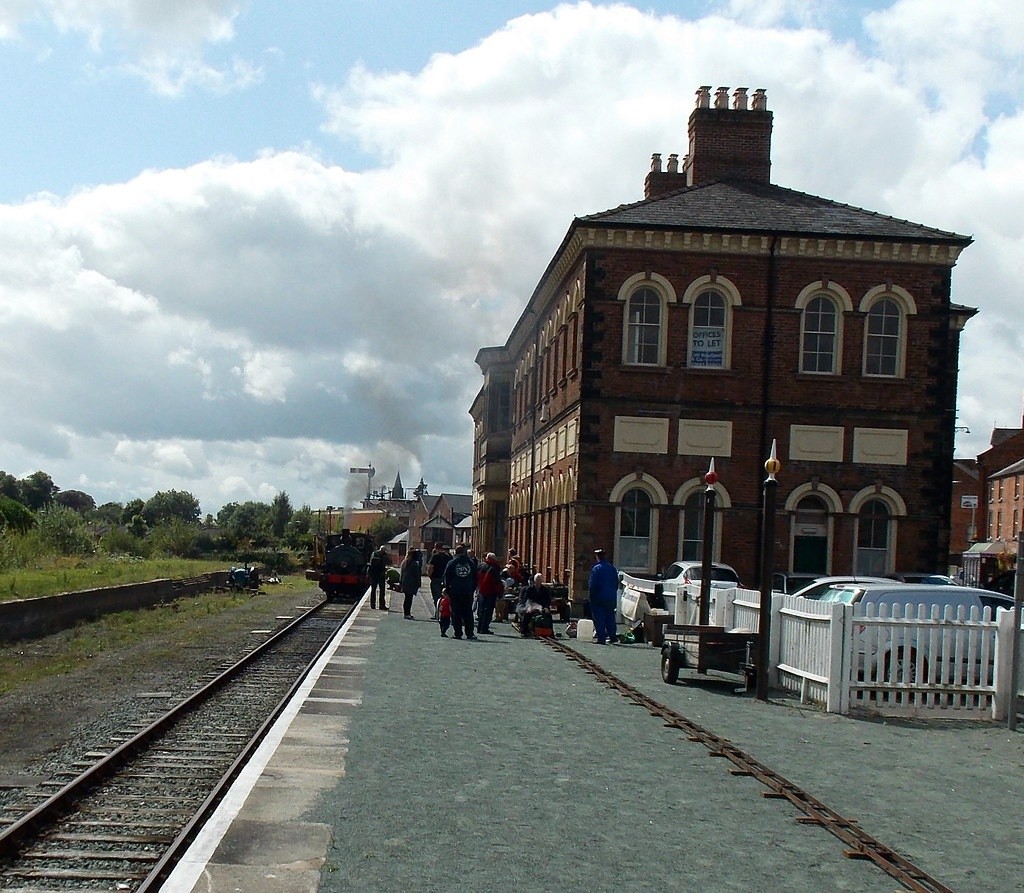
[656,560,745,590]
[758,570,831,593]
[880,572,958,586]
[789,576,904,599]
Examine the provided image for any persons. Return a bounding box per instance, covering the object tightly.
[493,548,521,622]
[443,546,476,639]
[589,550,618,644]
[439,588,451,637]
[519,573,555,639]
[384,567,400,591]
[476,552,504,634]
[399,546,423,619]
[426,540,453,620]
[367,545,389,610]
[457,543,478,566]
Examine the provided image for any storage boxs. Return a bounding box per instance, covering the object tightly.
[650,608,664,615]
[657,611,670,615]
[534,627,552,637]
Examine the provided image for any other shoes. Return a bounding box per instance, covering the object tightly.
[404,615,414,620]
[606,639,618,644]
[453,635,461,638]
[477,629,494,634]
[379,607,389,610]
[467,635,478,639]
[371,606,375,609]
[593,640,605,644]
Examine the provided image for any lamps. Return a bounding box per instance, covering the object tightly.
[539,404,552,423]
[511,482,518,488]
[542,465,554,474]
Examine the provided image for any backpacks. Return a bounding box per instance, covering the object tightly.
[368,551,384,578]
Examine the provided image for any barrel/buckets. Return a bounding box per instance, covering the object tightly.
[577,619,594,642]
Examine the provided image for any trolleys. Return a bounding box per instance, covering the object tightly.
[659,622,760,693]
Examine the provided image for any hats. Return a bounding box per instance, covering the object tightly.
[595,549,606,557]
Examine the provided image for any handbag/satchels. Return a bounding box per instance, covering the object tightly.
[472,590,479,613]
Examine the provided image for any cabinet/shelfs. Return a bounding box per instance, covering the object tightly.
[644,612,674,648]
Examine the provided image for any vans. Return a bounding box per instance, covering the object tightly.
[817,582,1024,704]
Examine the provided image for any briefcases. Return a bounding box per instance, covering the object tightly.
[534,609,553,637]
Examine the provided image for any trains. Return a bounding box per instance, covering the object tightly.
[305,527,378,604]
